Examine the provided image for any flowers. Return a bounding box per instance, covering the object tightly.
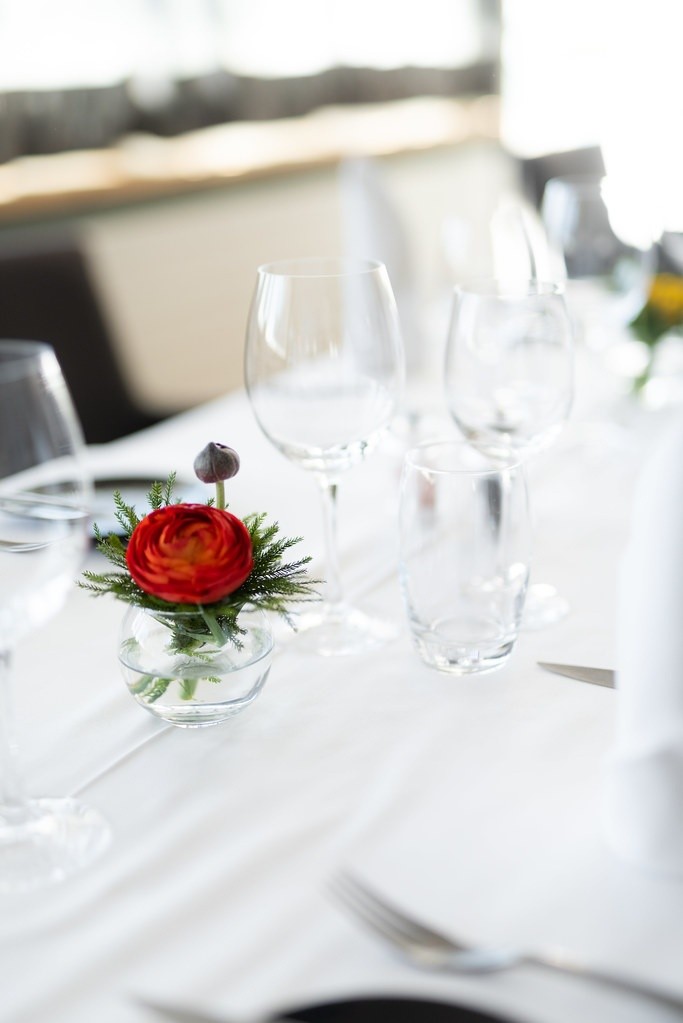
[69,438,325,699]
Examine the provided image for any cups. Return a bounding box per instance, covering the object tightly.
[400,442,529,677]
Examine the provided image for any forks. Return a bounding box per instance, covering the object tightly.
[326,867,683,1022]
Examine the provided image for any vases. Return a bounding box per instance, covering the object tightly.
[118,604,279,728]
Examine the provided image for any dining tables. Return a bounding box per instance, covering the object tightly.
[0,275,683,1023]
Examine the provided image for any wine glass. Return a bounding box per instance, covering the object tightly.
[0,338,115,889]
[443,279,578,622]
[241,254,406,658]
[540,174,656,450]
